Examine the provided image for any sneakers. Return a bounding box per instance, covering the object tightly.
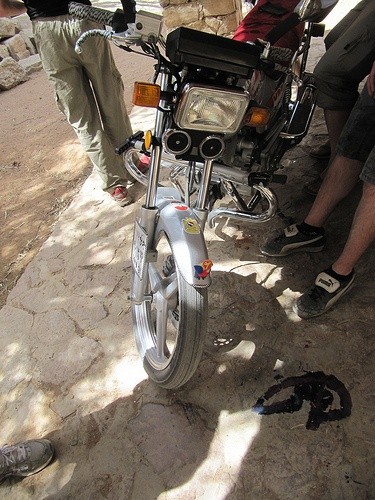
[259,221,324,257]
[125,154,150,185]
[0,439,54,483]
[293,264,356,318]
[111,187,130,206]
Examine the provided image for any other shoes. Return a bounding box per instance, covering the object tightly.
[310,139,330,157]
[306,176,362,196]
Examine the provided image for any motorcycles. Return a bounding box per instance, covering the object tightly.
[68,0,340,392]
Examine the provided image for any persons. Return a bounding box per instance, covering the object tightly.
[0,438,55,485]
[302,0,375,198]
[0,0,151,206]
[259,58,375,318]
